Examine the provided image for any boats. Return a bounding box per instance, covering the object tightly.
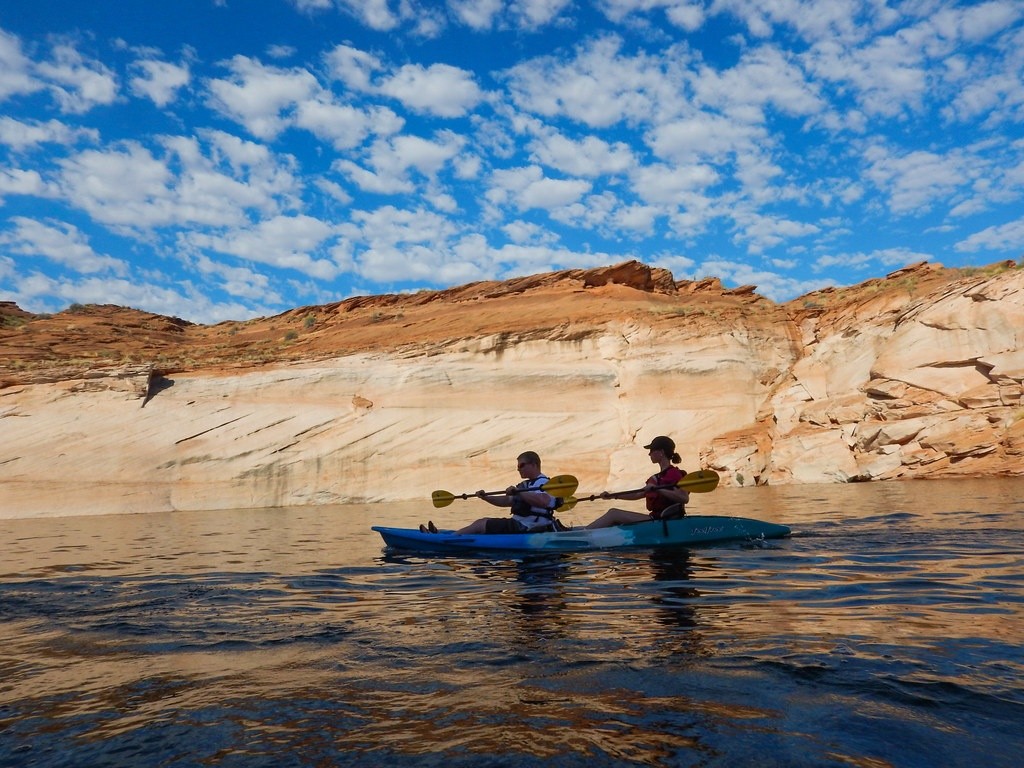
[369,514,794,552]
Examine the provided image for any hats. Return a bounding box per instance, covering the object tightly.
[644,436,675,450]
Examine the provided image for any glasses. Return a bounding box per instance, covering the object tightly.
[518,462,532,469]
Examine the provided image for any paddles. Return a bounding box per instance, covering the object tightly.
[554,469,720,513]
[431,475,579,508]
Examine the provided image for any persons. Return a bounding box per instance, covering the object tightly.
[419,451,555,535]
[552,436,689,532]
